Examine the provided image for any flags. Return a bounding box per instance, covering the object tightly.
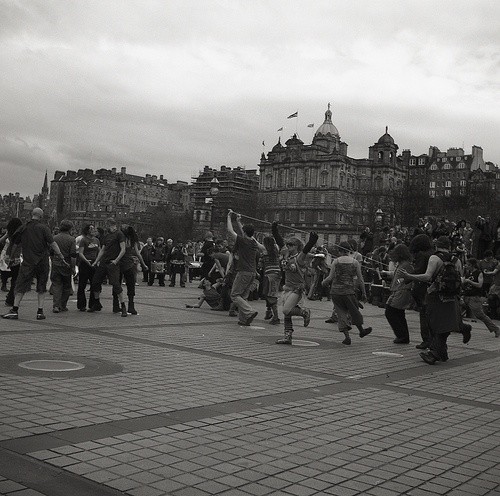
[277,128,283,131]
[287,112,298,118]
[262,141,265,146]
[307,124,314,128]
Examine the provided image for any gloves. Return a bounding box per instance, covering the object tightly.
[302,232,319,254]
[272,220,285,249]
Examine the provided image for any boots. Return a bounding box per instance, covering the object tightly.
[112,299,122,312]
[127,302,137,314]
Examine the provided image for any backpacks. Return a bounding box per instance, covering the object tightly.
[437,254,463,297]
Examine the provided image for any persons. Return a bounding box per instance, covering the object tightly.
[0,209,500,365]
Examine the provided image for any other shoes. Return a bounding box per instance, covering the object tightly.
[238,311,258,326]
[359,326,373,338]
[36,313,45,319]
[342,339,351,346]
[123,280,186,288]
[494,327,500,338]
[416,341,429,348]
[121,309,128,317]
[264,311,273,320]
[87,307,100,312]
[275,338,292,344]
[462,324,472,344]
[392,337,411,344]
[420,354,436,365]
[271,319,281,325]
[303,309,311,327]
[325,319,337,323]
[53,308,59,312]
[61,307,68,311]
[1,309,18,319]
[1,285,10,291]
